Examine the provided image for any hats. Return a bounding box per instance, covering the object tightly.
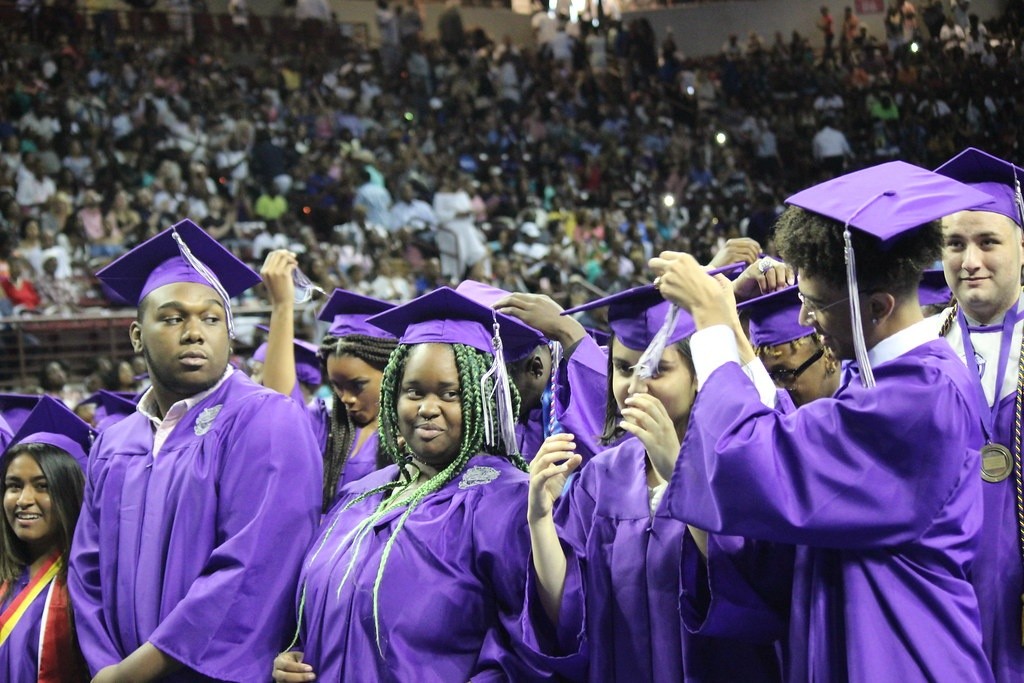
[253,321,324,388]
[315,287,404,338]
[93,386,140,432]
[934,146,1024,230]
[97,218,263,340]
[918,269,953,305]
[784,159,997,391]
[5,396,99,475]
[561,260,748,381]
[0,394,61,455]
[367,287,547,458]
[457,278,548,360]
[737,287,822,347]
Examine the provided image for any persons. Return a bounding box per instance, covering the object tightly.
[0,0,1024,429]
[0,148,1024,683]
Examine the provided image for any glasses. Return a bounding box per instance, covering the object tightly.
[768,351,825,383]
[796,291,872,322]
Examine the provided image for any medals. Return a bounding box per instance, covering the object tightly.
[980,443,1013,483]
